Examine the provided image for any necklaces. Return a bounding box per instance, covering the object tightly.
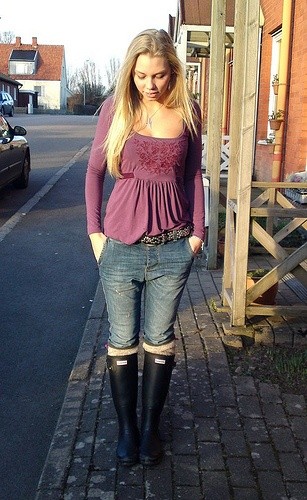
[138,97,165,127]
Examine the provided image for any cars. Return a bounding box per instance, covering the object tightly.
[0,112,31,189]
[0,91,14,117]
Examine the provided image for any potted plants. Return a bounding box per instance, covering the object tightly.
[267,109,285,130]
[247,268,278,303]
[271,74,279,95]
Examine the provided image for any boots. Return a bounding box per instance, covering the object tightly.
[138,339,177,467]
[105,345,140,466]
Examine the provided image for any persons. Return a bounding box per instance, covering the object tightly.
[83,28,206,468]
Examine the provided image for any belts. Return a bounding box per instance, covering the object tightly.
[138,224,192,245]
